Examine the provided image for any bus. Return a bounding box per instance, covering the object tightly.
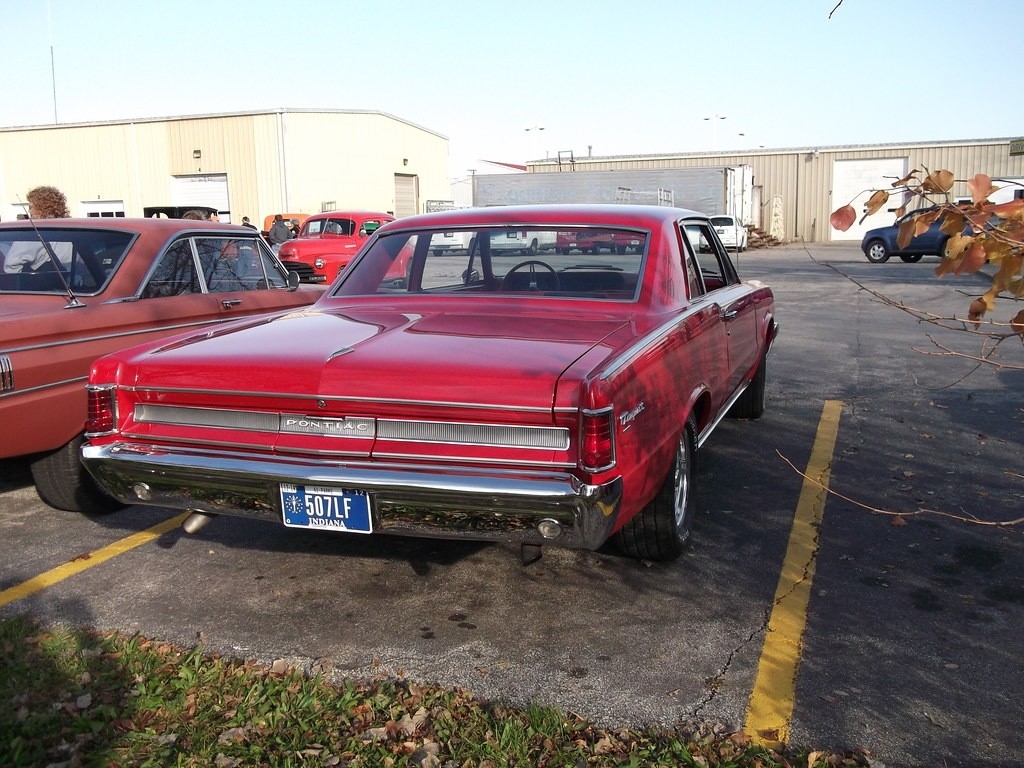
[863,206,1001,263]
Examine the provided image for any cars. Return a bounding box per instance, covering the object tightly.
[697,215,751,250]
[0,216,335,512]
[82,204,779,563]
[257,212,414,284]
[556,229,646,262]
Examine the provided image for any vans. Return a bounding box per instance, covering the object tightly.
[428,226,478,256]
[491,228,557,253]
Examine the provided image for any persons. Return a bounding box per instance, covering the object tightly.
[182,210,220,278]
[320,211,332,233]
[4,188,86,289]
[236,217,257,275]
[269,215,302,258]
